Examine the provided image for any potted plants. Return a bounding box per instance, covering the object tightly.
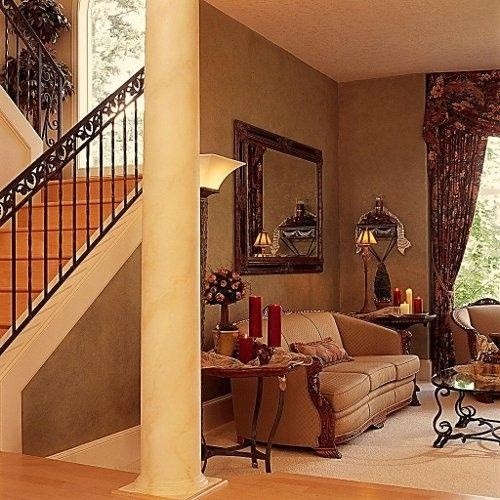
[372,260,392,309]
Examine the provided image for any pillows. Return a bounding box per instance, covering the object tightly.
[291,336,354,371]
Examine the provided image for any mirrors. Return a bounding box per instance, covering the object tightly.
[232,119,326,276]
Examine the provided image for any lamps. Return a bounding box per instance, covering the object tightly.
[252,233,273,254]
[355,227,377,314]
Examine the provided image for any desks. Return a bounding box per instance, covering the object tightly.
[352,311,437,391]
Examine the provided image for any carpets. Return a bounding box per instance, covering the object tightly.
[200,378,500,498]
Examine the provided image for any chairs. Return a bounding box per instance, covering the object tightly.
[449,298,500,403]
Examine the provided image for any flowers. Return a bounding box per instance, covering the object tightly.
[203,262,246,330]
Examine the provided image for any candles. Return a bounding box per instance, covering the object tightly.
[267,303,282,349]
[394,286,423,314]
[236,333,253,364]
[248,295,263,338]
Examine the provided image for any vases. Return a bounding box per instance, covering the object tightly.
[212,327,238,358]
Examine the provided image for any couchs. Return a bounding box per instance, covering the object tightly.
[230,308,422,458]
